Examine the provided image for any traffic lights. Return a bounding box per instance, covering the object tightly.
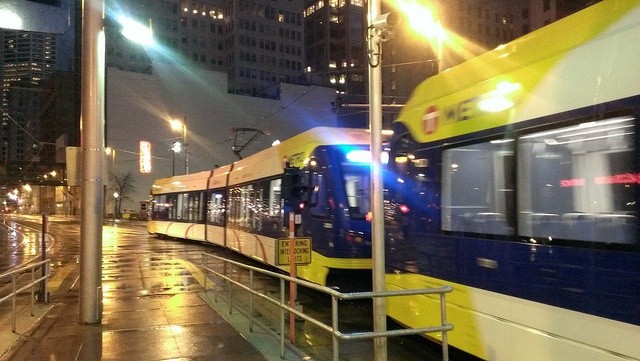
[140,140,151,173]
[285,167,308,214]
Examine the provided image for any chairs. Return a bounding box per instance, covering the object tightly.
[520,211,535,236]
[597,214,635,243]
[567,213,596,242]
[528,213,564,237]
[474,212,508,236]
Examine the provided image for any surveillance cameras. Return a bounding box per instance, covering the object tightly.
[373,12,401,29]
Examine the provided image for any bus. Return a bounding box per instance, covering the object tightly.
[147,126,393,293]
[385,0,640,360]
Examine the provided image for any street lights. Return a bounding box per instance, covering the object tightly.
[172,141,181,175]
[173,120,188,175]
[368,1,441,360]
